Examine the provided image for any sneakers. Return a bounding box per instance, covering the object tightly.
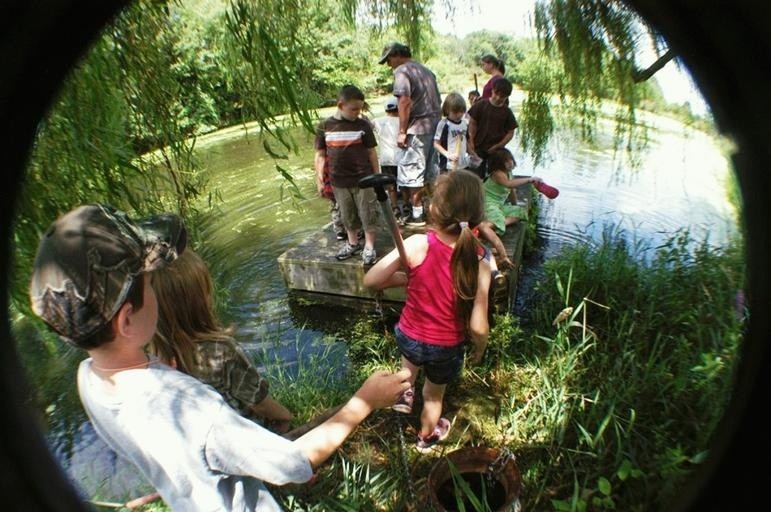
[392,207,401,224]
[402,204,409,220]
[392,386,415,415]
[403,214,426,226]
[337,233,345,240]
[360,246,376,265]
[416,418,451,455]
[358,235,362,239]
[335,240,362,260]
[492,271,508,305]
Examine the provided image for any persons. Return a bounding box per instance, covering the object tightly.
[147,246,293,436]
[29,201,413,512]
[364,170,492,453]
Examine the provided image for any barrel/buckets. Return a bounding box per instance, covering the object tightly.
[426,445,523,512]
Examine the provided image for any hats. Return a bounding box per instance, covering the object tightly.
[384,96,398,111]
[377,41,410,64]
[29,205,187,348]
